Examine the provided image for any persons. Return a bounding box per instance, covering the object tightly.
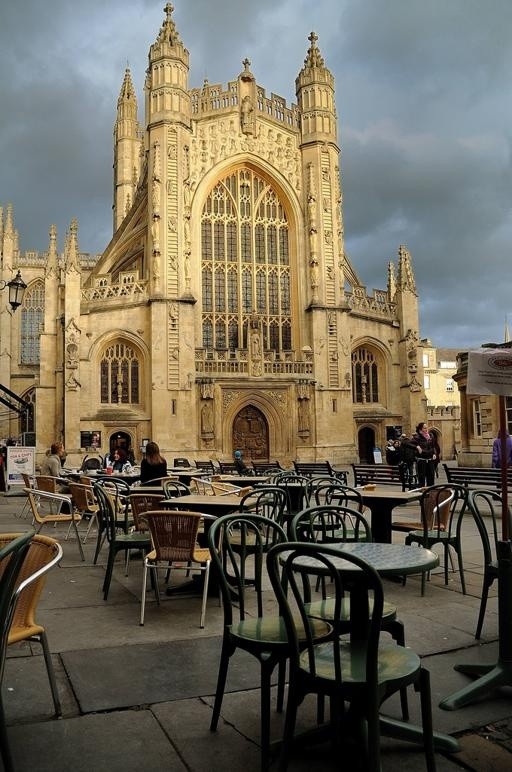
[129,442,168,496]
[384,422,441,492]
[491,428,512,502]
[233,451,255,475]
[0,435,138,521]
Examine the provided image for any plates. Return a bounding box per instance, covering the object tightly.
[88,473,97,474]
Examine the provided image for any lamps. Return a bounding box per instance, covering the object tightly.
[0,269,27,313]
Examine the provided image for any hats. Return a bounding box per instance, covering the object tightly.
[400,434,408,439]
[235,451,240,459]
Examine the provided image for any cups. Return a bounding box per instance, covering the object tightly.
[89,470,96,474]
[71,469,77,473]
[106,466,113,475]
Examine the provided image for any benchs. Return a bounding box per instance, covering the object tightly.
[174,458,423,507]
[442,464,512,494]
